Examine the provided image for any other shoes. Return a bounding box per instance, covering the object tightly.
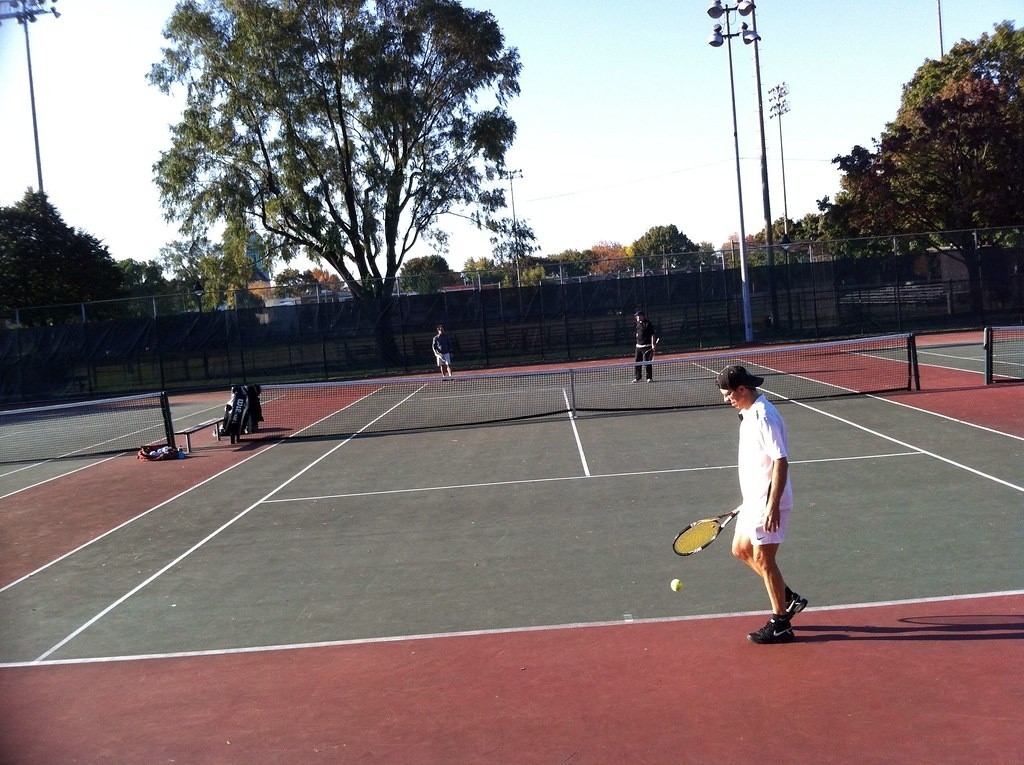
[646,378,653,383]
[632,378,642,383]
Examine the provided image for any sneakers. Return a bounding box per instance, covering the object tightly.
[785,592,808,622]
[747,616,796,644]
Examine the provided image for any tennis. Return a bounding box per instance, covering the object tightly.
[670,578,683,592]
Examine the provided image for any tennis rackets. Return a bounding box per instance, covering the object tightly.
[672,503,743,556]
[643,338,660,362]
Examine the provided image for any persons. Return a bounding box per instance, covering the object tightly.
[432,325,452,381]
[631,311,655,384]
[715,365,808,645]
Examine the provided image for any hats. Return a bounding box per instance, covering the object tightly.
[716,364,764,390]
[634,310,645,316]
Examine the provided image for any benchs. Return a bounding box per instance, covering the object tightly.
[172,418,224,453]
[838,282,946,308]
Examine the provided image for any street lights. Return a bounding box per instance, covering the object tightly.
[499,165,525,322]
[778,234,793,334]
[705,0,763,346]
[0,0,62,203]
[767,81,792,247]
[191,279,210,379]
